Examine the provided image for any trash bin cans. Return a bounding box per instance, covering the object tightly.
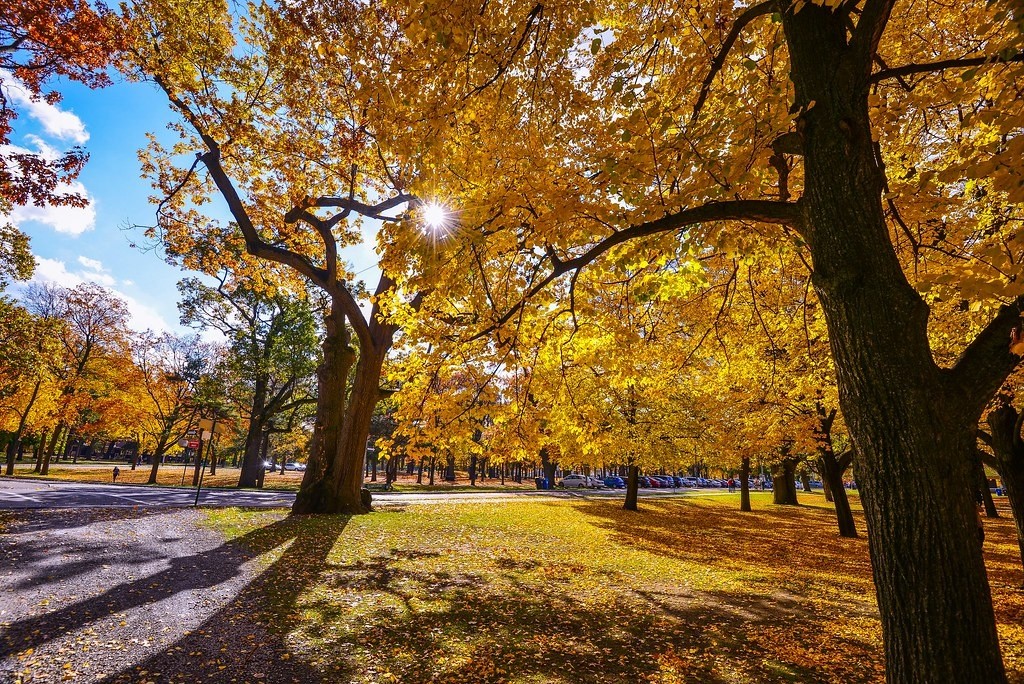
[535,477,544,490]
[995,488,1002,496]
[1003,489,1008,496]
[543,477,549,489]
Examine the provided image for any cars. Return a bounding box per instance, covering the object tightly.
[809,480,822,488]
[794,480,803,490]
[847,480,857,489]
[168,455,182,462]
[266,463,282,470]
[557,474,774,488]
[285,463,306,471]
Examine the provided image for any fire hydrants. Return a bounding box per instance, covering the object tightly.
[112,466,119,483]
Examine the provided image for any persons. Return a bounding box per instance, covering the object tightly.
[727,477,734,493]
[137,456,143,466]
[112,466,120,483]
[753,477,767,491]
